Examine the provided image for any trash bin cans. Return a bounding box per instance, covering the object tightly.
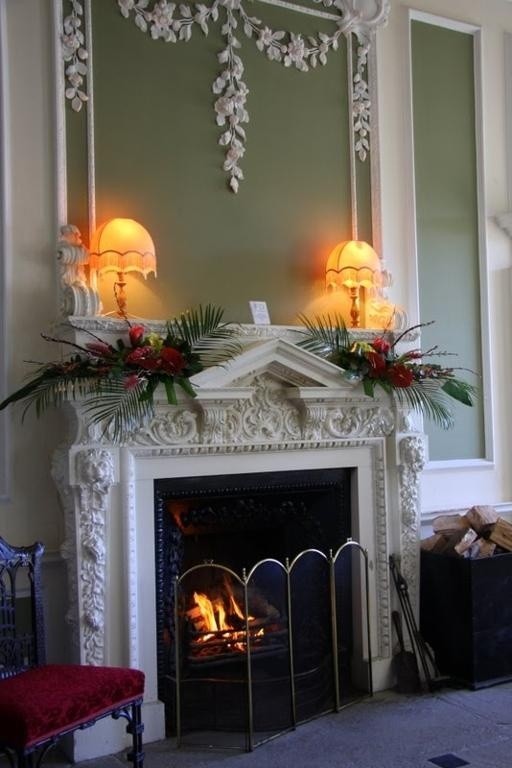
[421,538,511,690]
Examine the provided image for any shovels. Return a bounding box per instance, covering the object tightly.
[392,611,419,691]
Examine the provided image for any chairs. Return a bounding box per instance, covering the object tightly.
[0,536,146,768]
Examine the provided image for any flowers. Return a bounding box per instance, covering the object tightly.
[288,312,482,431]
[0,301,244,442]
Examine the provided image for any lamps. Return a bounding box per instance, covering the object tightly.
[95,215,158,319]
[325,241,379,329]
